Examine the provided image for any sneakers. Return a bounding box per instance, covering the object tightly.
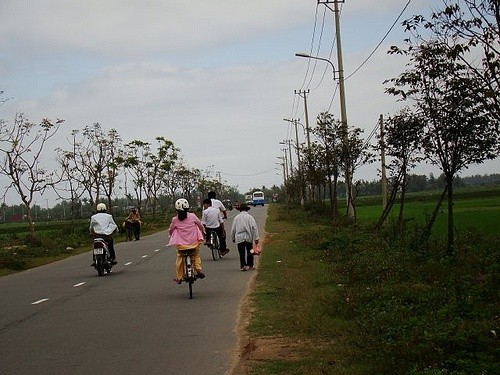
[221,249,229,256]
[241,266,247,271]
[204,242,211,246]
[248,265,255,270]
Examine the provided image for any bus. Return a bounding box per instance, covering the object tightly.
[253,189,264,207]
[272,193,280,203]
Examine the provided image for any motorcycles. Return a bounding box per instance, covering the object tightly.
[176,249,203,296]
[228,204,233,211]
[88,219,118,279]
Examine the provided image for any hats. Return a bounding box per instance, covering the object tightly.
[236,203,251,210]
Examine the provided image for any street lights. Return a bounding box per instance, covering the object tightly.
[296,52,355,217]
[276,162,283,175]
[280,140,293,177]
[277,156,286,180]
[285,118,313,167]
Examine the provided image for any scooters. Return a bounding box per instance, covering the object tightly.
[235,203,239,210]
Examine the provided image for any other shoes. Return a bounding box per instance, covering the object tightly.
[196,272,205,279]
[177,279,182,284]
[90,262,95,267]
[110,262,118,265]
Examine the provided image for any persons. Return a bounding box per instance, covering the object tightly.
[124,207,142,241]
[230,202,260,271]
[89,202,120,266]
[168,197,207,284]
[202,190,230,254]
[202,198,226,255]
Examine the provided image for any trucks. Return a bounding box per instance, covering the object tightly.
[245,194,252,198]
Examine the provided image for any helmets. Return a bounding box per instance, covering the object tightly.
[175,198,189,211]
[97,203,106,211]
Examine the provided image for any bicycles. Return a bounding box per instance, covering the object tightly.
[124,219,133,242]
[205,229,224,260]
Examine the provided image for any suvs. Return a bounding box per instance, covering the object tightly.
[245,199,252,205]
